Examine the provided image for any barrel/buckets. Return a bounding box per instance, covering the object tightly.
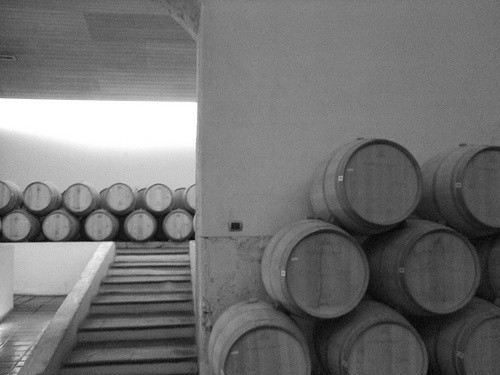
[408,143,499,240]
[357,217,481,318]
[260,218,370,321]
[207,299,312,375]
[0,179,196,243]
[469,234,500,308]
[412,295,500,375]
[308,137,424,236]
[313,298,429,375]
